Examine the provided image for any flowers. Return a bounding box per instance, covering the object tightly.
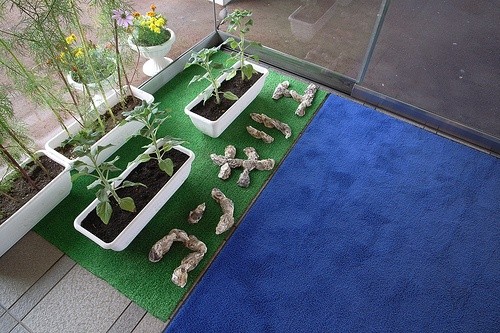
[45,33,107,82]
[111,3,169,45]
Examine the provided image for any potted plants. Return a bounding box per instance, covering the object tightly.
[0,85,73,257]
[0,0,156,174]
[185,7,269,139]
[69,99,196,251]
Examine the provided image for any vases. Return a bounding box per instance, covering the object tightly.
[287,0,339,43]
[128,27,176,76]
[66,56,118,109]
